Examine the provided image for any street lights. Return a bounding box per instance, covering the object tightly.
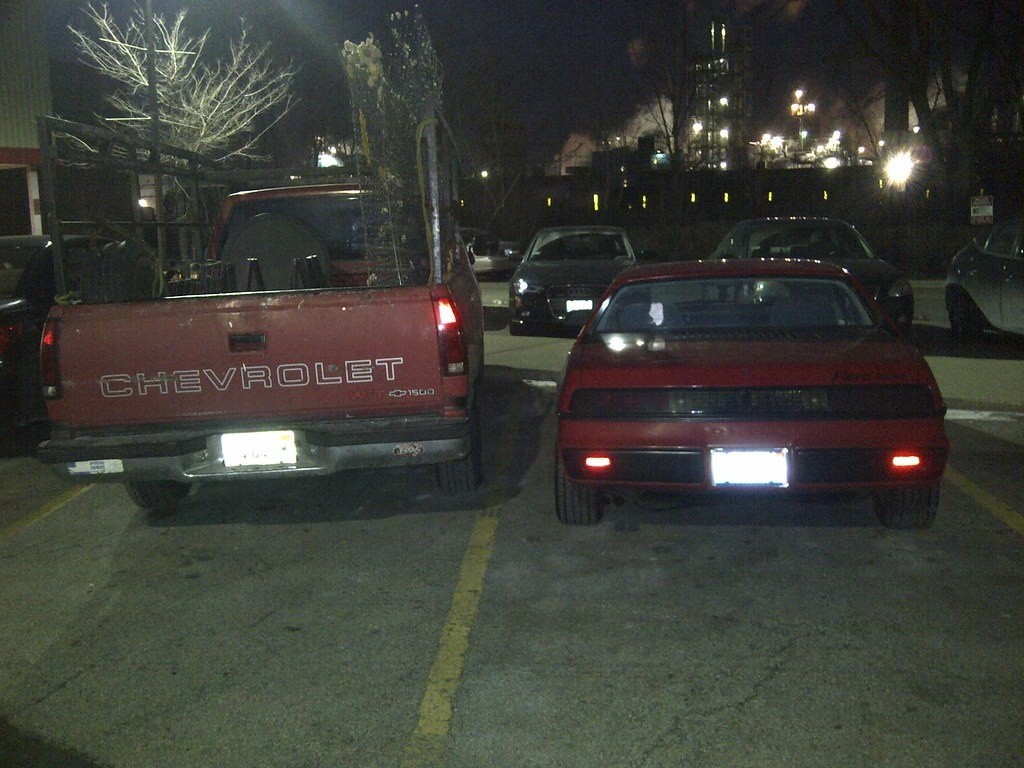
[789,89,816,170]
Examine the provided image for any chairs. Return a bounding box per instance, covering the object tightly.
[541,238,569,257]
[765,293,840,326]
[624,301,688,330]
[598,239,617,257]
[805,241,842,260]
[749,237,790,258]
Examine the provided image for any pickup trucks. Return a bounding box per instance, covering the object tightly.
[33,183,500,500]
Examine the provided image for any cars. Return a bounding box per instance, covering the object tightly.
[553,260,948,534]
[0,233,164,462]
[508,225,655,340]
[704,216,915,340]
[944,210,1024,343]
